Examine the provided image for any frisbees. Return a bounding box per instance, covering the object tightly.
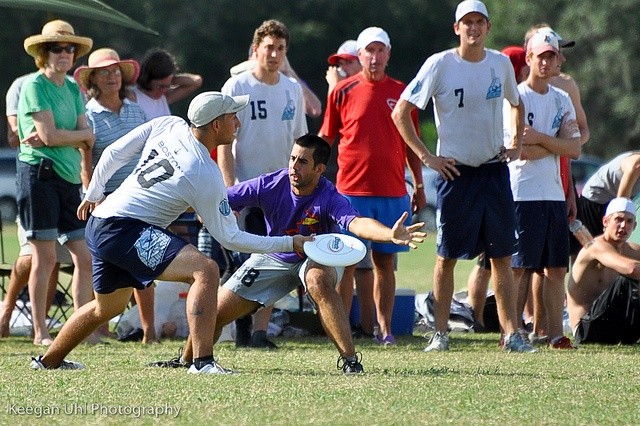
[302,232,368,268]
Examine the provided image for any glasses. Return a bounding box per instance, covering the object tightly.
[47,44,75,53]
[95,68,121,76]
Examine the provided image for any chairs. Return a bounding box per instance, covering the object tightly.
[0,210,72,332]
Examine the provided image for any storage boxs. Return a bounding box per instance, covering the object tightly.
[349,288,415,335]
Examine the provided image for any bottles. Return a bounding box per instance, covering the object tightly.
[567,216,596,250]
[168,292,189,339]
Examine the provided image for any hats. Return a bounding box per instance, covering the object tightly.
[73,49,140,93]
[455,0,488,22]
[24,21,93,59]
[526,32,560,59]
[524,27,576,48]
[328,40,358,64]
[605,197,637,217]
[357,27,390,50]
[187,91,249,127]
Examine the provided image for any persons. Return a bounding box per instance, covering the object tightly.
[123,50,201,119]
[503,34,580,347]
[17,20,111,348]
[31,91,316,373]
[570,151,640,267]
[216,20,309,346]
[2,44,76,337]
[81,49,158,346]
[325,39,373,338]
[147,134,426,375]
[567,197,640,343]
[462,23,589,341]
[391,0,532,351]
[318,26,426,346]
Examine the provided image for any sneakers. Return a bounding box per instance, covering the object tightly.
[354,323,379,344]
[380,335,396,347]
[145,347,192,368]
[550,337,577,349]
[423,330,450,352]
[249,342,278,349]
[337,352,364,374]
[187,357,233,375]
[529,333,549,346]
[521,321,534,333]
[502,328,541,353]
[517,330,529,344]
[31,356,86,370]
[45,318,62,328]
